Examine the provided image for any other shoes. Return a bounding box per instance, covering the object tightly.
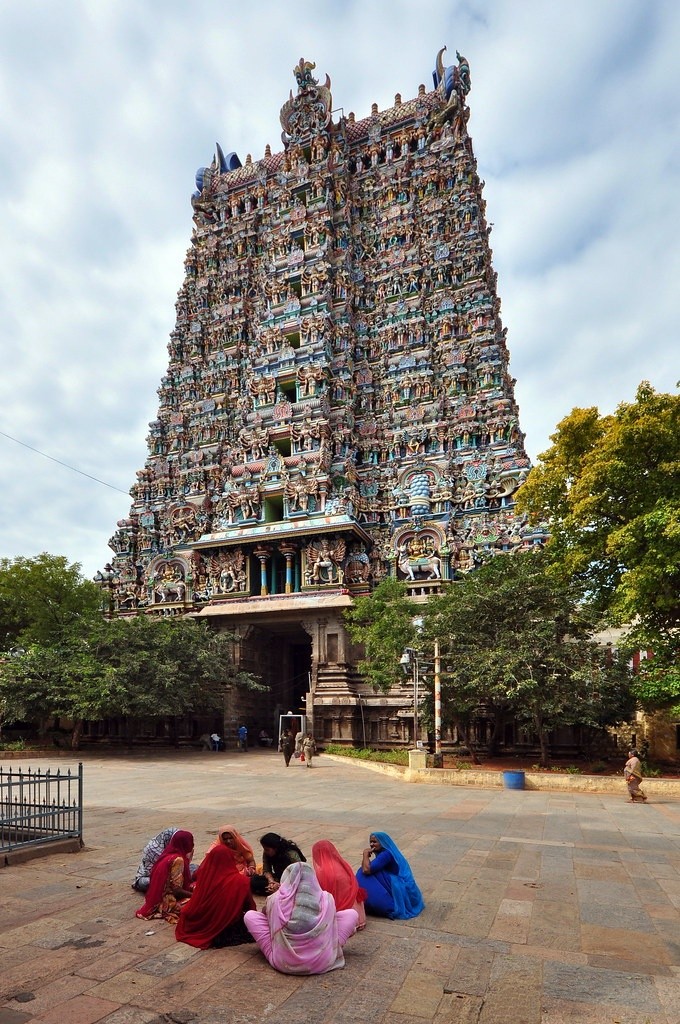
[240,750,242,753]
[627,800,635,803]
[287,764,289,767]
[307,763,312,768]
[641,798,648,804]
[244,749,248,752]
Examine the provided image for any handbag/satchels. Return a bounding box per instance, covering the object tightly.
[301,752,305,761]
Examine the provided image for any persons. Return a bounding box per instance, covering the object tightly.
[624,750,648,804]
[238,724,248,752]
[94,46,564,618]
[201,732,224,752]
[258,728,268,746]
[280,729,317,768]
[131,827,425,976]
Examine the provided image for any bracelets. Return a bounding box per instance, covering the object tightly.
[266,875,272,878]
